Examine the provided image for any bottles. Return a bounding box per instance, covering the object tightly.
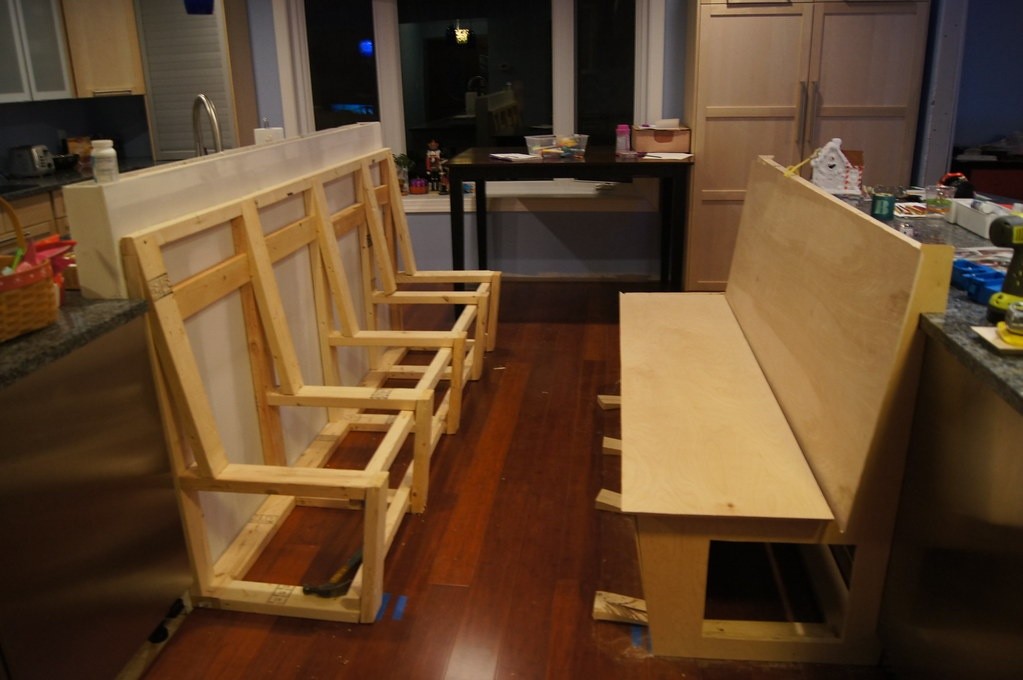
[615,124,630,155]
[91,140,120,183]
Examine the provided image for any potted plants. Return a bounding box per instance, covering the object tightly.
[393,150,414,196]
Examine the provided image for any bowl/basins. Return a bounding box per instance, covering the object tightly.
[53,153,80,171]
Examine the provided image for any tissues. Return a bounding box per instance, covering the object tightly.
[631,119,691,153]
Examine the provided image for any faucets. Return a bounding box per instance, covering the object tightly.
[192,94,222,155]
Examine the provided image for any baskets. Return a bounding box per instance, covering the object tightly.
[0,198,58,343]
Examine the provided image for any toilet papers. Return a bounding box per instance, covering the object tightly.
[253,127,284,145]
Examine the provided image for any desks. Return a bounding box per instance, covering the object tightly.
[443,143,694,324]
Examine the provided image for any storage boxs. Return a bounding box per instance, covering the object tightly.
[634,123,691,154]
[524,133,589,163]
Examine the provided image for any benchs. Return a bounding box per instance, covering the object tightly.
[118,148,501,622]
[618,154,956,672]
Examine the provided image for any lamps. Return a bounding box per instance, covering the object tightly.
[254,117,285,145]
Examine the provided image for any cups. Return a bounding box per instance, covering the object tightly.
[871,186,896,220]
[926,185,957,227]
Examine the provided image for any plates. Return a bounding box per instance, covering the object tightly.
[893,202,945,218]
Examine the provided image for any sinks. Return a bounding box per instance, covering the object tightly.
[0,182,39,196]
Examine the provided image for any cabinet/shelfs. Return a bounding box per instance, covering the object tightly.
[680,0,931,293]
[0,0,259,167]
